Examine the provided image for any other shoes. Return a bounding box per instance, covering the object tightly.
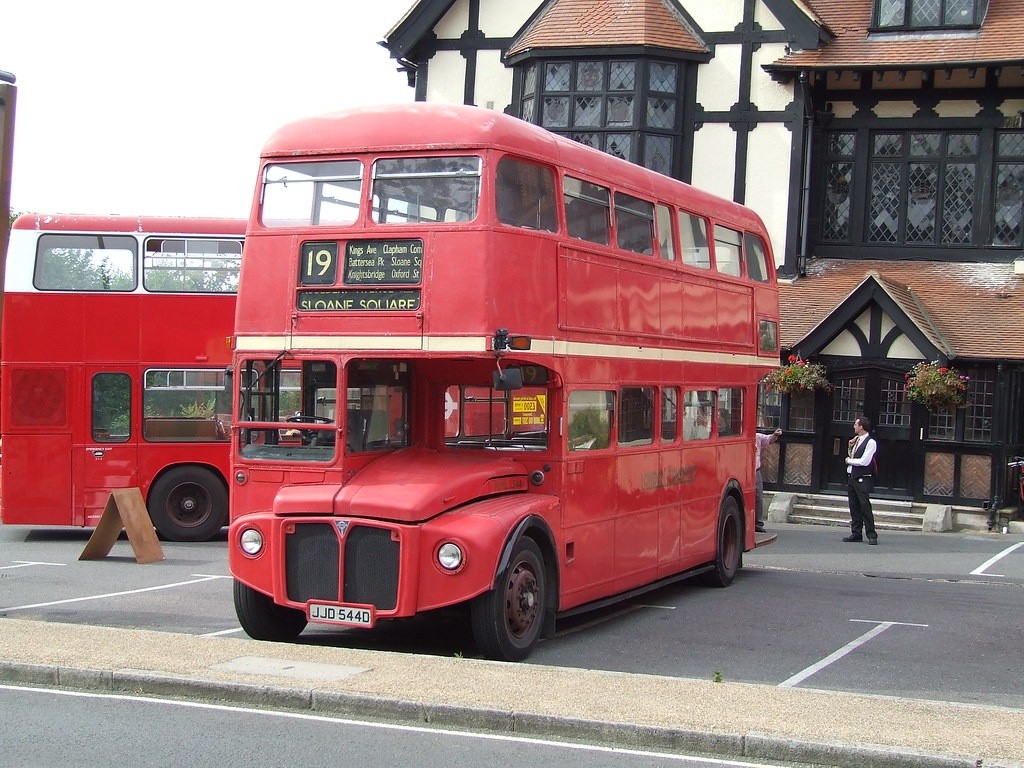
[842,536,863,542]
[869,537,877,545]
[755,525,766,533]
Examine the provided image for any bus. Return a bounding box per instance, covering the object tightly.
[228,102,782,663]
[1,212,544,543]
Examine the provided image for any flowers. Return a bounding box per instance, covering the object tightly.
[761,356,838,397]
[900,360,970,412]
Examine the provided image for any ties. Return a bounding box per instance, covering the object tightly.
[851,438,859,459]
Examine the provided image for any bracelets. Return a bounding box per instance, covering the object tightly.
[773,433,777,436]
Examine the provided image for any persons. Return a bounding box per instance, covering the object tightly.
[718,409,731,429]
[842,417,878,545]
[755,429,782,532]
